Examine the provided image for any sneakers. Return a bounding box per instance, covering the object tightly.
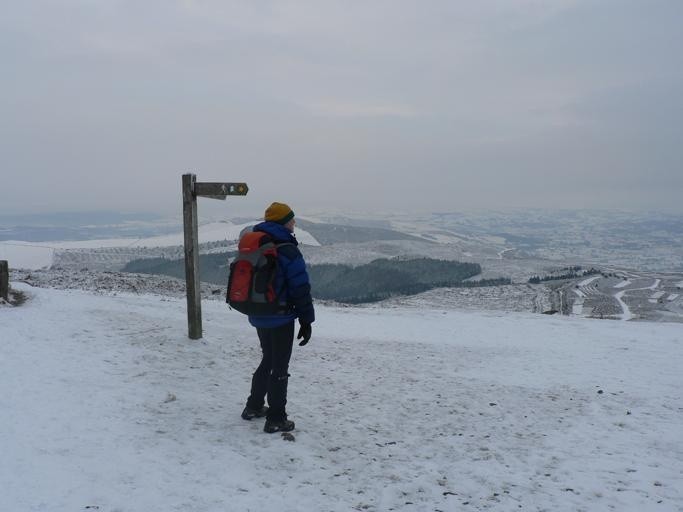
[239,406,272,420]
[263,413,295,433]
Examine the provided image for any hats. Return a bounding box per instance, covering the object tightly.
[263,201,295,226]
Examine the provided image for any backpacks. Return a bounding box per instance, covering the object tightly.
[224,231,297,318]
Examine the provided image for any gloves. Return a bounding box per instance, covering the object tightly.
[296,323,311,347]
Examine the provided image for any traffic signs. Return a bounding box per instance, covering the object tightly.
[194,182,248,201]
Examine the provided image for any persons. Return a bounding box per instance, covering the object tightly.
[225,203,315,434]
